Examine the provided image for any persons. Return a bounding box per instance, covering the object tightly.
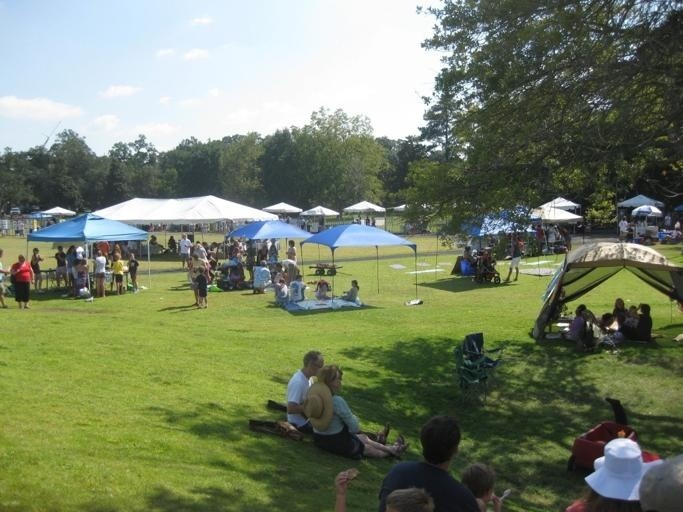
[122,222,194,270]
[461,234,524,284]
[460,459,502,511]
[333,469,435,512]
[530,223,572,255]
[311,366,405,461]
[187,238,330,309]
[342,279,360,302]
[1,245,141,308]
[567,298,653,350]
[284,351,390,445]
[617,216,629,242]
[567,438,645,511]
[375,414,482,511]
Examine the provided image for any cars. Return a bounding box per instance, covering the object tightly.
[403,221,432,234]
[9,206,20,215]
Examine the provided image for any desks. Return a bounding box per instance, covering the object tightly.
[33,264,133,297]
[209,260,286,283]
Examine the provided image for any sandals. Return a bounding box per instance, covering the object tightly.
[393,434,408,457]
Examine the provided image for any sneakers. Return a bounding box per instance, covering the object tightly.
[379,424,389,444]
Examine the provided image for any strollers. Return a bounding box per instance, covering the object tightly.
[482,260,501,285]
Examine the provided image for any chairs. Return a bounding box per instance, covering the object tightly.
[453,346,486,403]
[462,330,501,387]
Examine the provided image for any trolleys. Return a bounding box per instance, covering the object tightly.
[307,262,343,276]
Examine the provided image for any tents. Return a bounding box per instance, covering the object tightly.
[224,199,432,297]
[530,242,683,342]
[25,192,279,261]
[461,196,586,248]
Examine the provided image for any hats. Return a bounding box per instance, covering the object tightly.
[584,437,665,501]
[303,382,334,432]
